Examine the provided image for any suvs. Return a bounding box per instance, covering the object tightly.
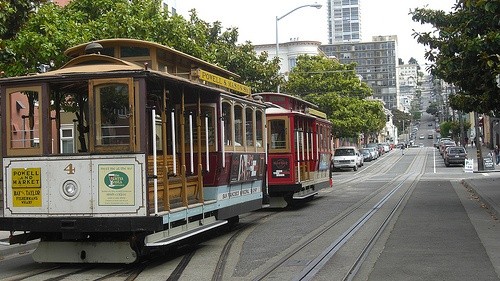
[331,146,361,173]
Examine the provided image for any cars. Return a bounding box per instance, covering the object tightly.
[356,141,390,165]
[409,119,425,147]
[428,112,468,167]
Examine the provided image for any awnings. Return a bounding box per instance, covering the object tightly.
[17,101,26,109]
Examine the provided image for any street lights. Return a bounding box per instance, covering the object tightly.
[275,3,323,93]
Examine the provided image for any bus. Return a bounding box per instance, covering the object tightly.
[244,91,333,202]
[0,37,271,264]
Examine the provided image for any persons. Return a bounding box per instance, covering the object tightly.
[495,144,500,166]
[401,144,405,155]
[464,135,476,148]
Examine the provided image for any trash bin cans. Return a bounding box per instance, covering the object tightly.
[482,156,495,170]
